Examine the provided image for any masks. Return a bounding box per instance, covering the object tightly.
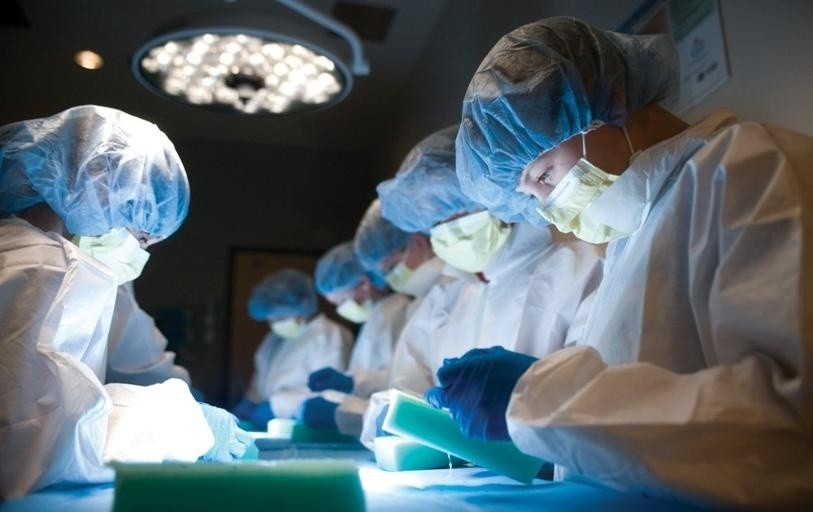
[425,209,508,273]
[384,255,439,298]
[335,296,375,325]
[535,121,643,246]
[72,229,151,287]
[268,318,308,341]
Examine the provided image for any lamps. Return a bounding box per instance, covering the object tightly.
[131,0,372,120]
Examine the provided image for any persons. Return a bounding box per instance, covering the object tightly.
[376,123,606,389]
[301,241,410,428]
[308,198,476,399]
[425,13,813,512]
[234,269,355,429]
[0,101,258,498]
